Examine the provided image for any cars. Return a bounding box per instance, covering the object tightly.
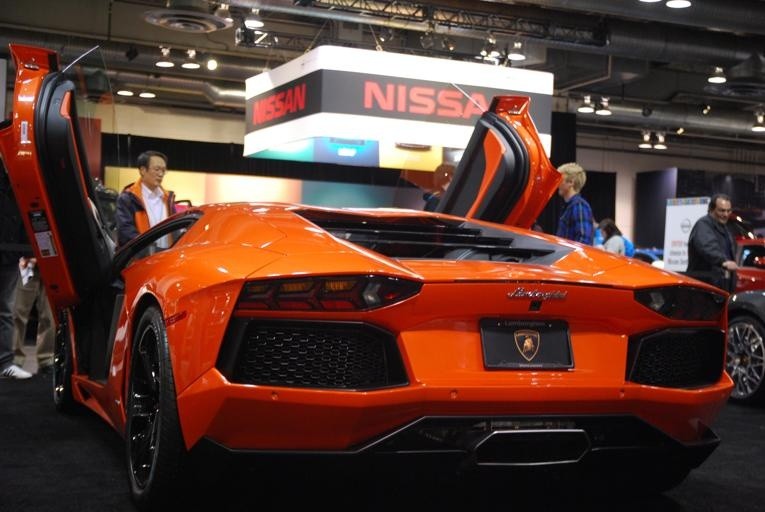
[727,212,765,405]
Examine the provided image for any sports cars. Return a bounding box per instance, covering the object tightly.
[1,41,736,510]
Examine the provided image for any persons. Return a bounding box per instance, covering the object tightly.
[555,162,594,246]
[423,164,455,212]
[17,256,56,375]
[685,194,740,294]
[597,218,627,254]
[116,150,181,260]
[0,119,32,380]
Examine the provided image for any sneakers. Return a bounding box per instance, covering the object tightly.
[0,364,33,380]
[38,364,54,380]
